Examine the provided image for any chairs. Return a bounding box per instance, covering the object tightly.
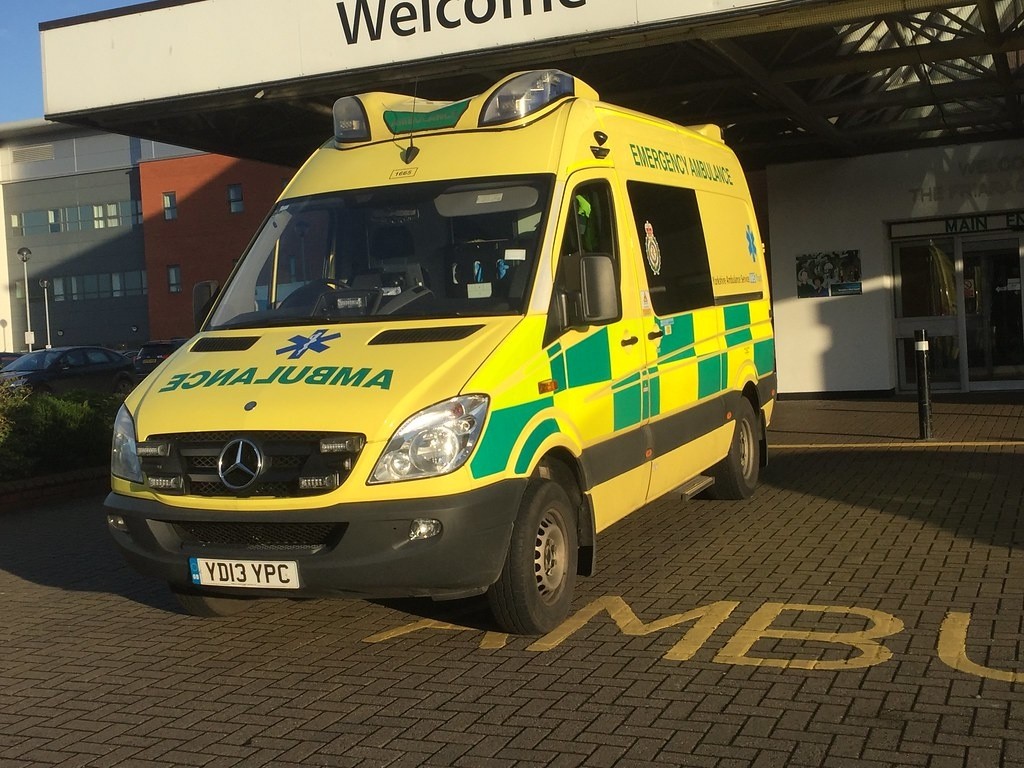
[349,227,428,297]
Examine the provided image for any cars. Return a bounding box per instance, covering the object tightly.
[2,345,181,404]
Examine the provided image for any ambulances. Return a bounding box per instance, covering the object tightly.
[100,68,777,637]
